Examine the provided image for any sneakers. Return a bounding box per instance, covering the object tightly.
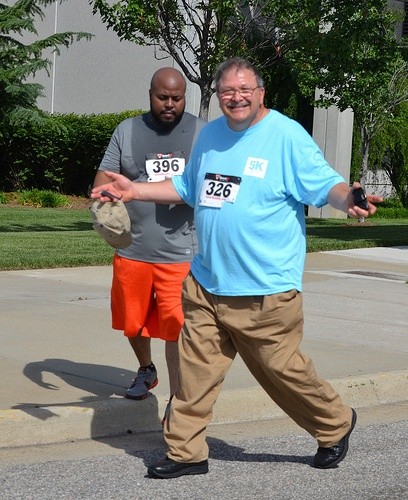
[125,361,158,400]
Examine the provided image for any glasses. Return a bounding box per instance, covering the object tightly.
[217,86,260,97]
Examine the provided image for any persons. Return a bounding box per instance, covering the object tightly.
[93,67,208,400]
[90,57,378,479]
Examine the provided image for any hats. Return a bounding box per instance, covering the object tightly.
[90,191,131,249]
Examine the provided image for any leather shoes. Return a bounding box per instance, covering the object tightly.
[148,455,208,479]
[313,408,357,468]
